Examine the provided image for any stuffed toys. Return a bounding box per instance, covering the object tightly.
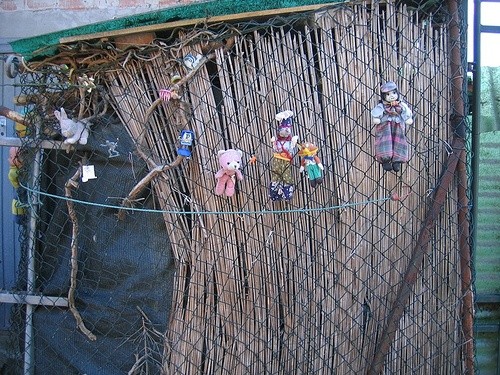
[268,110,301,202]
[297,142,325,186]
[216,148,244,197]
[53,106,89,145]
[7,145,28,224]
[371,79,414,171]
[158,52,204,104]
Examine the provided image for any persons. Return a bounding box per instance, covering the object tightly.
[100,140,120,156]
